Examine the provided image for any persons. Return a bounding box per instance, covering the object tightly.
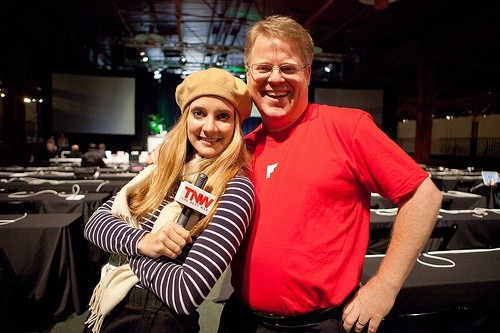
[81,143,107,168]
[38,131,69,161]
[68,145,84,158]
[217,15,444,333]
[82,68,256,333]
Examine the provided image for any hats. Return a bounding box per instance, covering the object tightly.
[174,67,254,125]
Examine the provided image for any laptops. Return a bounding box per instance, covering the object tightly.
[481,171,500,187]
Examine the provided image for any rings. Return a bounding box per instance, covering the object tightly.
[358,320,366,327]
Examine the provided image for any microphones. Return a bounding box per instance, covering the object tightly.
[174,173,216,228]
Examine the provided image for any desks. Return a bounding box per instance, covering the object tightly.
[147,134,164,153]
[358,165,500,333]
[0,162,151,333]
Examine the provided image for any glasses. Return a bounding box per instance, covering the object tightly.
[249,62,308,75]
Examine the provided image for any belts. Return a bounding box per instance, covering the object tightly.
[241,286,358,331]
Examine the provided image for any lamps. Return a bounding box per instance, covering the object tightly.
[325,64,333,72]
